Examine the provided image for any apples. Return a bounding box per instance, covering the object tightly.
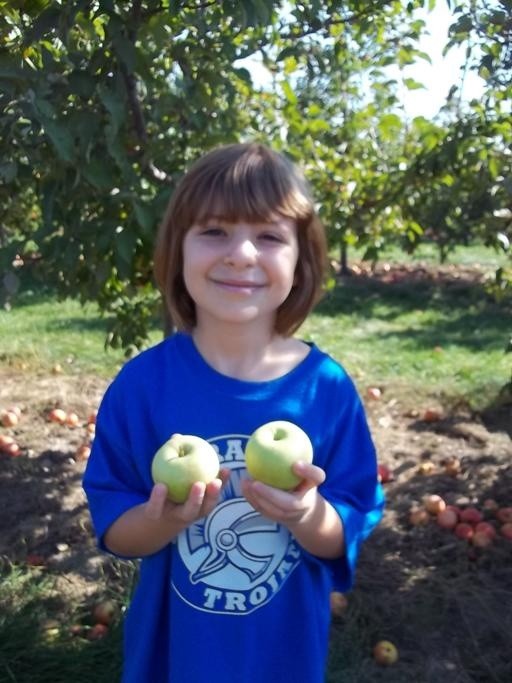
[152,434,219,504]
[374,640,398,664]
[28,555,44,565]
[410,494,511,548]
[245,421,313,489]
[1,403,99,461]
[424,408,437,422]
[379,461,394,481]
[70,600,116,641]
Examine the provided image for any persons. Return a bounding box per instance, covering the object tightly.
[80,140,384,683]
